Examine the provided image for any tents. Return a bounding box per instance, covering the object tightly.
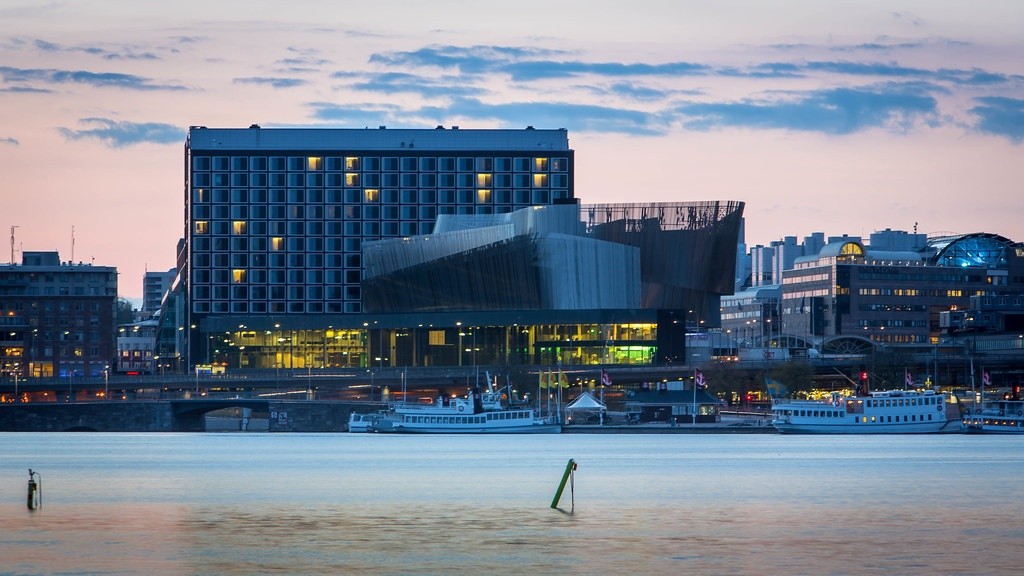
[566,391,606,426]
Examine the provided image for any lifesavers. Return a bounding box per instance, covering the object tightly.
[835,401,840,406]
[775,400,779,405]
[976,409,982,415]
[1018,410,1022,416]
[964,409,970,415]
[999,410,1004,416]
[937,405,942,411]
[459,406,464,411]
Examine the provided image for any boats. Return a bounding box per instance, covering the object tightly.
[960,385,1024,435]
[770,371,982,435]
[347,370,562,434]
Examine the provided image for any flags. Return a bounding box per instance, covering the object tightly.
[558,369,568,389]
[601,367,612,386]
[765,379,789,395]
[905,369,914,385]
[549,369,557,389]
[695,369,706,386]
[982,371,992,385]
[539,370,548,389]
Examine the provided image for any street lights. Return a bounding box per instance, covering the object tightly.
[272,362,281,399]
[104,365,110,401]
[304,364,314,400]
[69,368,77,403]
[158,364,170,399]
